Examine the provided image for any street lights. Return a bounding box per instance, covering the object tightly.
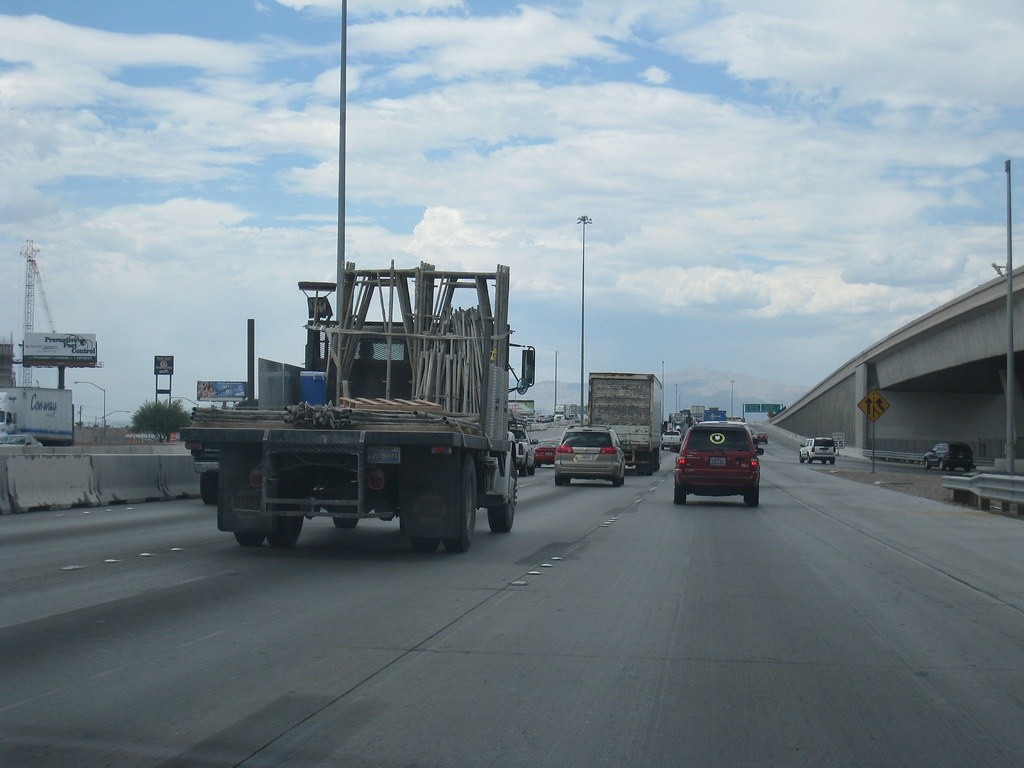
[73,380,106,441]
[575,214,594,425]
[102,410,130,432]
[730,380,735,423]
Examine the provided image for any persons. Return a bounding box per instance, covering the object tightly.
[359,342,376,360]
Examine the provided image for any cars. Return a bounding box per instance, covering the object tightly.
[535,439,561,467]
[523,415,579,424]
[755,432,769,444]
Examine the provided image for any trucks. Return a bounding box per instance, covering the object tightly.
[180,264,536,548]
[666,406,727,438]
[587,372,663,477]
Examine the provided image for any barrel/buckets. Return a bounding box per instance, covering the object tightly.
[299,371,327,406]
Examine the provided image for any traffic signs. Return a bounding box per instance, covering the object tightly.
[745,403,761,413]
[761,403,781,413]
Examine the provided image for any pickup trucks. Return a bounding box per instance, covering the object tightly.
[508,425,540,476]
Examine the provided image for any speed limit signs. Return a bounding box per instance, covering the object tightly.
[832,433,845,449]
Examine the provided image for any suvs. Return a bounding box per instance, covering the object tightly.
[673,421,765,506]
[553,423,626,486]
[923,442,974,471]
[661,430,681,450]
[799,437,836,465]
